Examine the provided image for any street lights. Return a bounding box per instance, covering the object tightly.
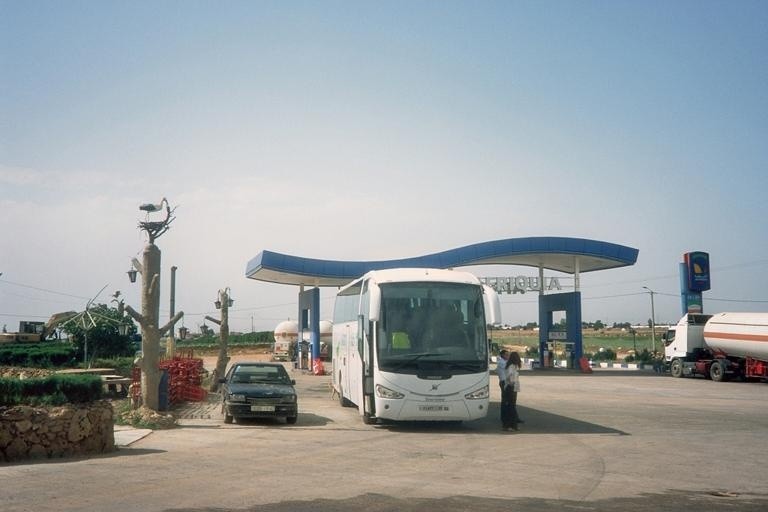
[642,284,656,356]
[198,286,234,387]
[125,254,190,411]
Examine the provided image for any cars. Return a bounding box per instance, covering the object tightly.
[217,361,300,427]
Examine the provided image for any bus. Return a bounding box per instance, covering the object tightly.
[328,265,505,430]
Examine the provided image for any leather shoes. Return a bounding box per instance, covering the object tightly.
[501,417,526,431]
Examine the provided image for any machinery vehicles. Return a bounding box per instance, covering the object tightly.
[661,309,768,384]
[270,316,333,360]
[0,301,107,344]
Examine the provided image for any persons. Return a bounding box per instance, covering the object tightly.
[496,349,526,431]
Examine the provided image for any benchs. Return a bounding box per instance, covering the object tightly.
[103,376,134,396]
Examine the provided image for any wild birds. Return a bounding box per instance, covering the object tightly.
[139,197,169,223]
